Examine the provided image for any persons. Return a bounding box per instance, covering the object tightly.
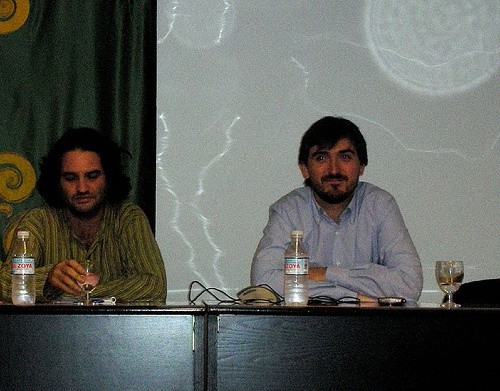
[0,126,167,307]
[250,115,424,307]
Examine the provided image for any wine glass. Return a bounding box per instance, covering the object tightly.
[74,259,100,305]
[436,261,464,308]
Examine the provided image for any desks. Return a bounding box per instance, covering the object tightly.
[1,301,206,391]
[203,300,500,391]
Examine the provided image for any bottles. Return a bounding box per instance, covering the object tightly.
[11,230,35,306]
[283,231,309,306]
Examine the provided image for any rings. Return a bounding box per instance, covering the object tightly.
[65,258,72,266]
